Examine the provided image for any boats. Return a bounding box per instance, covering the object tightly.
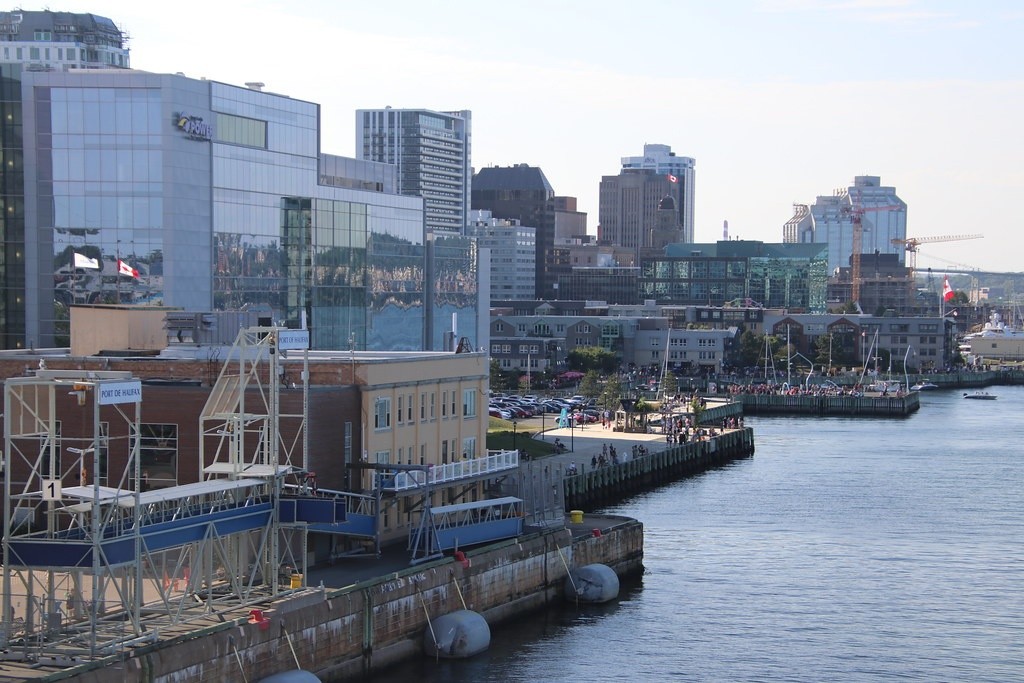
[911,379,938,391]
[962,391,998,399]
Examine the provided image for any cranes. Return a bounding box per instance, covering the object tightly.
[843,189,904,313]
[890,235,986,312]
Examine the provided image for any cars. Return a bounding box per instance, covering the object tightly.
[488,390,563,420]
[554,396,604,424]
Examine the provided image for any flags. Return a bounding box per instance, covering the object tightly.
[117,259,139,279]
[942,276,954,303]
[73,253,99,268]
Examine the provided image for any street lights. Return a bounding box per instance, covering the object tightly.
[512,420,518,450]
[499,373,502,393]
[661,402,667,437]
[630,398,636,433]
[569,403,576,453]
[690,375,694,398]
[675,375,680,398]
[604,391,607,411]
[542,403,546,441]
[581,402,586,432]
[654,367,658,392]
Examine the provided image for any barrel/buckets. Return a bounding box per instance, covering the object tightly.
[290,574,303,589]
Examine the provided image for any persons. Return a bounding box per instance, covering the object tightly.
[517,382,902,476]
